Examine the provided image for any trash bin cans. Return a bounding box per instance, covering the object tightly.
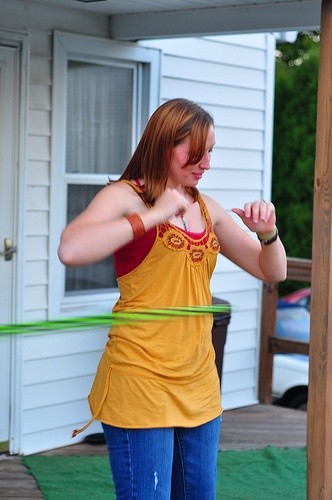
[211,296,231,395]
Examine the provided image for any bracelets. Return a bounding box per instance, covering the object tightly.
[257,225,278,246]
[122,211,145,242]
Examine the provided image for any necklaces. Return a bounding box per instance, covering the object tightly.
[180,214,188,232]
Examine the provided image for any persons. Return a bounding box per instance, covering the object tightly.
[57,98,288,500]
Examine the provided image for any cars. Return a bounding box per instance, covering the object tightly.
[268,284,312,412]
